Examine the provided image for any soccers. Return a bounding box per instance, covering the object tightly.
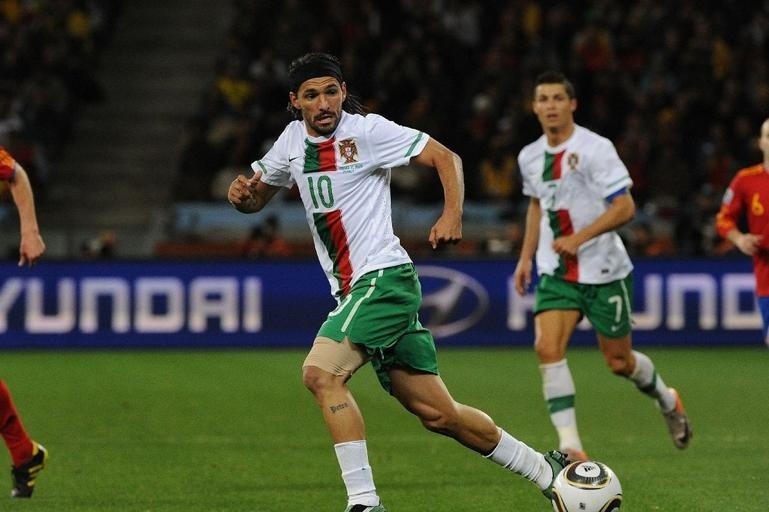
[551,460,624,512]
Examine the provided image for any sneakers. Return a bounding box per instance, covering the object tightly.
[11,441,49,498]
[541,450,572,503]
[658,387,693,450]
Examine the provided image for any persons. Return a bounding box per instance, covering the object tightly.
[0,149,49,497]
[1,1,113,194]
[716,120,769,347]
[158,1,769,265]
[228,52,569,512]
[513,69,691,463]
[84,230,118,265]
